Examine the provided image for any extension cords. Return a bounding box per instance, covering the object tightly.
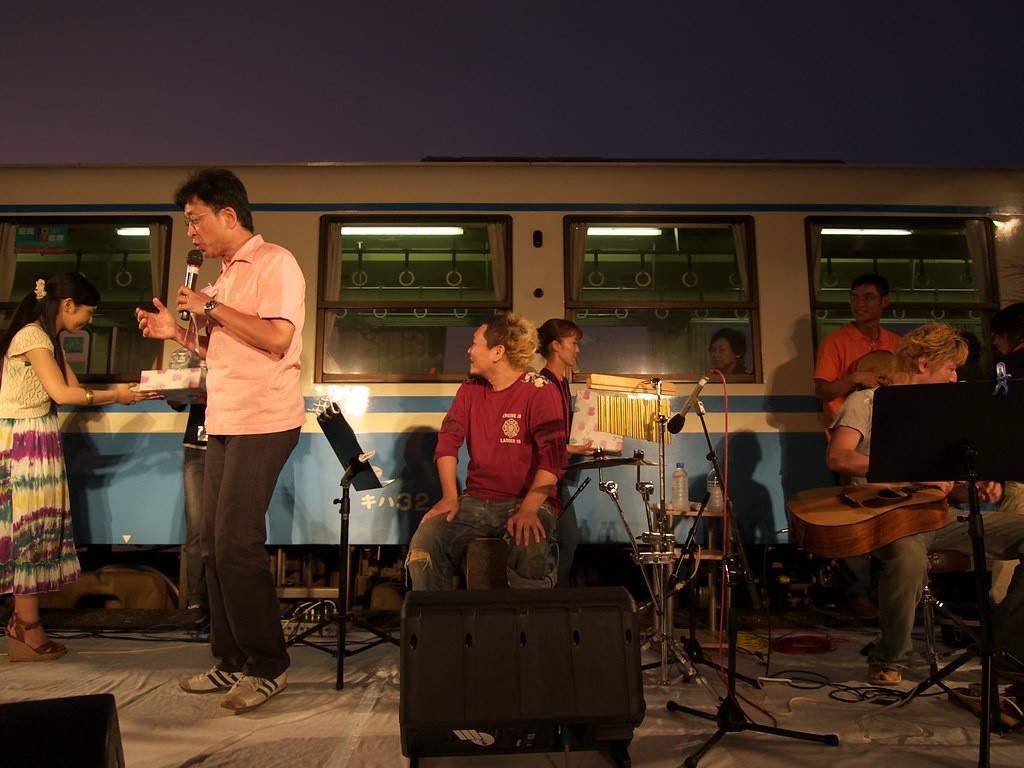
[281,619,338,635]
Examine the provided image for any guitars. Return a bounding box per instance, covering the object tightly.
[784,480,990,559]
[853,349,895,390]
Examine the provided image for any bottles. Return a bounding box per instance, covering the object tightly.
[706,464,724,512]
[672,462,689,510]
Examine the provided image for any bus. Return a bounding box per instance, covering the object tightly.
[0,157,1024,562]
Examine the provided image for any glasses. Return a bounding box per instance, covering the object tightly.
[184,206,216,226]
[847,293,881,303]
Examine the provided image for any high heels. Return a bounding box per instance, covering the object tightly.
[4,611,67,664]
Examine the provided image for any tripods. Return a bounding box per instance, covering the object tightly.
[597,379,840,768]
[865,378,1024,768]
[284,403,400,690]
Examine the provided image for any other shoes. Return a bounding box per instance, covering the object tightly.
[868,666,902,685]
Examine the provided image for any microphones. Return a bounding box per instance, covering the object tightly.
[668,369,714,434]
[180,250,203,321]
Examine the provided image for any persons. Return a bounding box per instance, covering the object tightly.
[535,319,596,588]
[0,270,166,662]
[826,321,1001,685]
[811,272,904,618]
[975,301,1024,609]
[136,165,307,710]
[708,328,754,375]
[407,314,570,590]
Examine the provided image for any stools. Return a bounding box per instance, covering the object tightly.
[885,550,960,698]
[647,504,740,645]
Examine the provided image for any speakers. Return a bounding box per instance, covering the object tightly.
[399,585,646,768]
[0,693,126,768]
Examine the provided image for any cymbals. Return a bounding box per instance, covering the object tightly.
[561,457,638,470]
[622,459,660,467]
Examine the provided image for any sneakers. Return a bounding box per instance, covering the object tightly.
[178,665,243,693]
[219,668,288,710]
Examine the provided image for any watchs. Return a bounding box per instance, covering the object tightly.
[204,300,223,323]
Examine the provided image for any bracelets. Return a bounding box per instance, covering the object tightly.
[82,386,93,407]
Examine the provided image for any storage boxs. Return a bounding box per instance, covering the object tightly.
[140,368,206,405]
